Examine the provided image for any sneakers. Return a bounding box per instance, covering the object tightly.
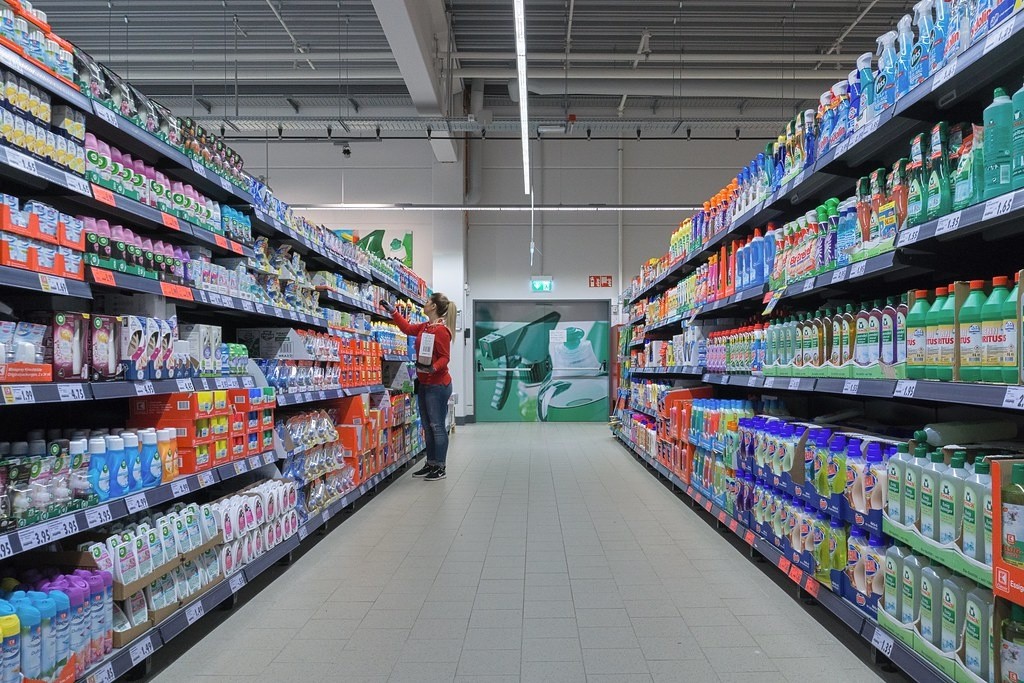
[424,465,446,480]
[412,463,433,477]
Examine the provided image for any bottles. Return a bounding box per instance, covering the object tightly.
[368,253,404,277]
[1,425,177,521]
[0,568,114,683]
[370,297,430,355]
[618,86,1024,683]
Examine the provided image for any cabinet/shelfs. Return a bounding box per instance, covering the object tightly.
[609,0,1024,683]
[0,3,435,683]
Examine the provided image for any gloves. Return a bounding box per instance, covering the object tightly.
[379,299,395,313]
[415,360,434,373]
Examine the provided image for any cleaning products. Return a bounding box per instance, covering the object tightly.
[0,0,457,678]
[613,2,1024,678]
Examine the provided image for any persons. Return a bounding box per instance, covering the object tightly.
[380,293,457,481]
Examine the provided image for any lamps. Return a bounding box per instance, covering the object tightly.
[263,131,705,213]
[511,0,532,195]
